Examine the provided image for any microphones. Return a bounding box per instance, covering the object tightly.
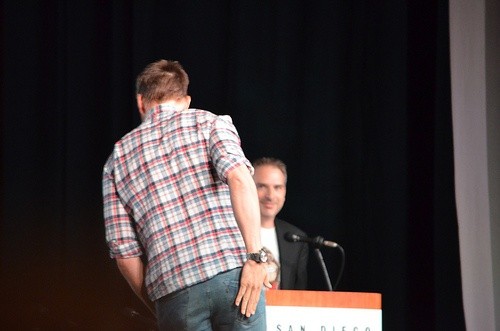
[283,230,338,249]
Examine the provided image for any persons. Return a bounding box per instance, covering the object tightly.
[102,59,273,331]
[252,157,308,291]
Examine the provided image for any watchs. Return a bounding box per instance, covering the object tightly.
[247,249,268,264]
